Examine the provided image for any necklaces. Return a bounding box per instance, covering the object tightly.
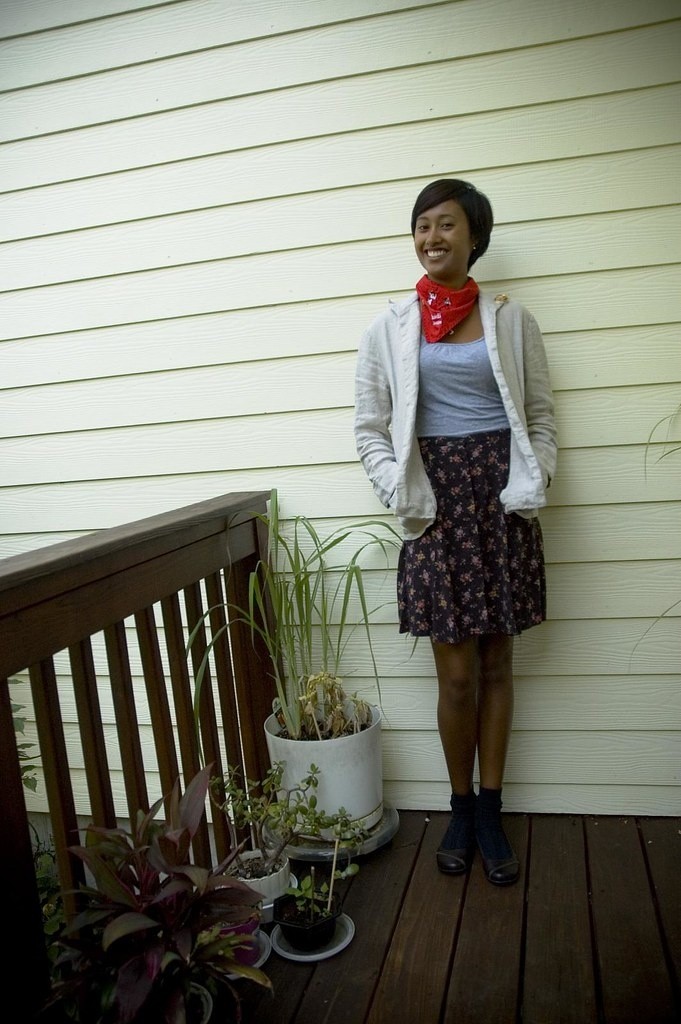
[448,329,454,335]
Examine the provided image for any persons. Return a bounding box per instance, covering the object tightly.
[354,178,560,885]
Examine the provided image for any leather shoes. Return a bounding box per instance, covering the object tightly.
[435,818,478,876]
[477,830,520,887]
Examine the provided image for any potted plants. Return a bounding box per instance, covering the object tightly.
[184,488,419,863]
[207,760,368,924]
[207,900,272,980]
[56,759,275,1024]
[270,867,355,961]
